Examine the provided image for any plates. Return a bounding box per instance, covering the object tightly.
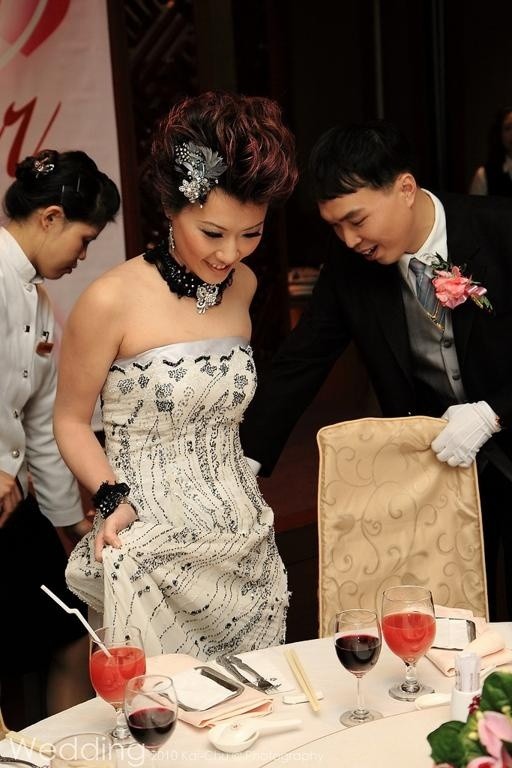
[414,693,451,709]
[209,723,258,754]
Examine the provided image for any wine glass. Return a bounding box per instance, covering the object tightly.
[88,626,146,747]
[381,585,438,702]
[335,610,384,728]
[124,673,178,768]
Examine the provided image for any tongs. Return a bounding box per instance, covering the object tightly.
[217,653,279,700]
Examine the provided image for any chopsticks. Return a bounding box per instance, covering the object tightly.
[3,730,52,757]
[281,646,323,714]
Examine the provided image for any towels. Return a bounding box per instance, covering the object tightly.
[138,653,274,729]
[405,603,511,676]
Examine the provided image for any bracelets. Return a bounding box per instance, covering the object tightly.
[90,481,131,518]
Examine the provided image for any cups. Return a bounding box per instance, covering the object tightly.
[50,732,118,768]
[454,685,482,723]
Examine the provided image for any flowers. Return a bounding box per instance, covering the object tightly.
[426,670,512,768]
[421,251,495,311]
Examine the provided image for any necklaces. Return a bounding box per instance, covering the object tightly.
[139,241,236,315]
[424,300,444,332]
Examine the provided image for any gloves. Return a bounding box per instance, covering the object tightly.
[428,399,504,472]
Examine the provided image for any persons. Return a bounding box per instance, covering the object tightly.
[238,115,510,623]
[51,89,301,698]
[0,145,123,729]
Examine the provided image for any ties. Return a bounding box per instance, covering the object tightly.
[405,259,445,329]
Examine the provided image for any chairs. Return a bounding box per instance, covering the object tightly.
[315,416,490,642]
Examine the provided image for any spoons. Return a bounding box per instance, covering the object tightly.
[221,718,303,743]
